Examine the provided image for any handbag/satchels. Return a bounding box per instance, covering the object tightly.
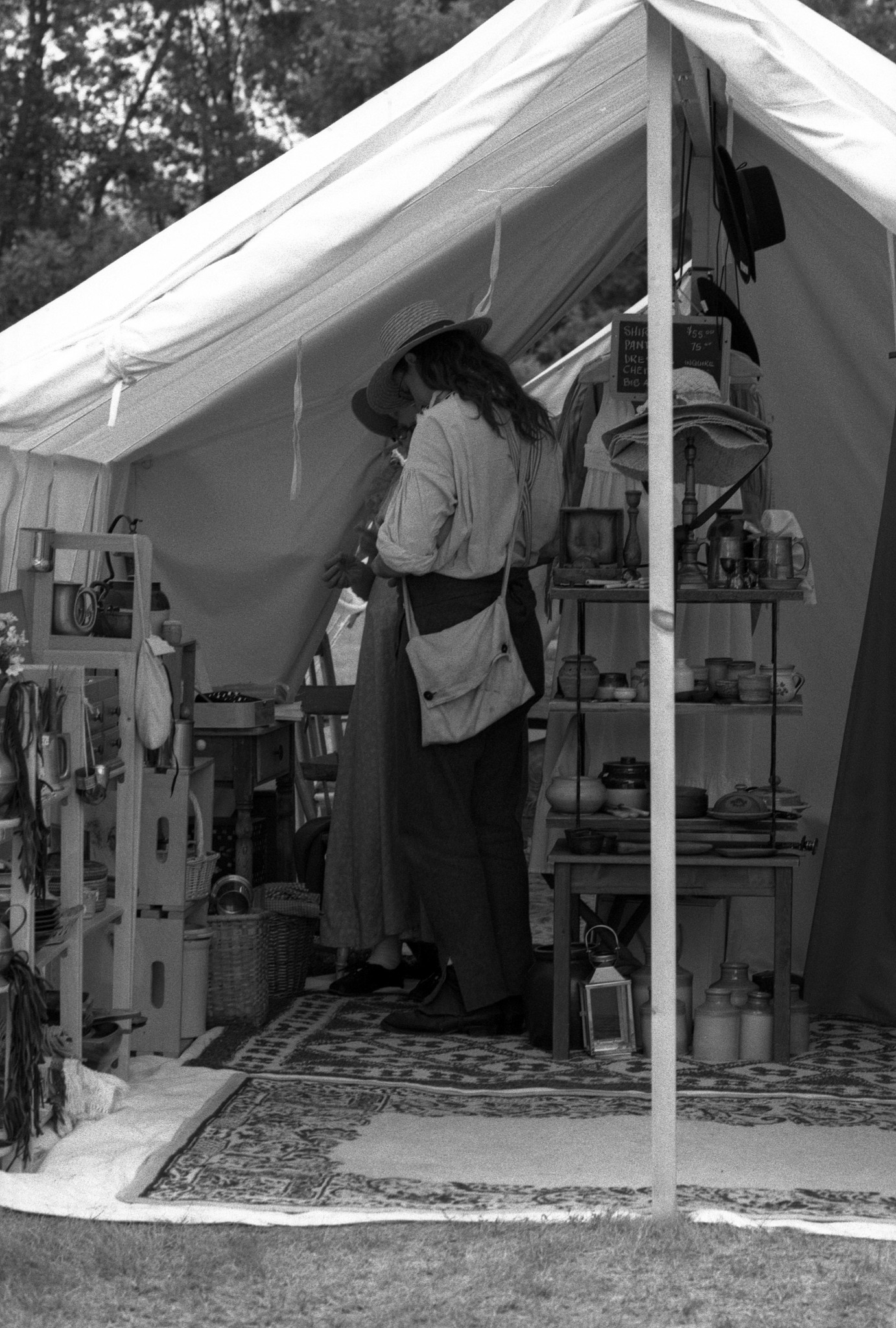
[135,634,176,751]
[405,595,536,747]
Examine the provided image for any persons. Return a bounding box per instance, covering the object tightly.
[322,298,566,1040]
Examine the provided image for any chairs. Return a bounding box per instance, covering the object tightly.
[294,631,356,833]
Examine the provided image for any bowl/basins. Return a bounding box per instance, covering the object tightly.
[546,776,607,817]
[106,611,132,638]
[1,899,62,952]
[675,794,708,817]
[614,687,637,702]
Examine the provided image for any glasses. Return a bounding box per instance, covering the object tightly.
[398,370,413,401]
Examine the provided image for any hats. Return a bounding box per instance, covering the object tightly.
[697,276,761,382]
[367,298,492,415]
[601,366,773,487]
[717,143,787,283]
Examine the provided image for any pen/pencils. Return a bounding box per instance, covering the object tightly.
[355,520,373,557]
[586,576,649,589]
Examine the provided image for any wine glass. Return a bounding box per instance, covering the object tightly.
[744,559,768,590]
[718,557,741,589]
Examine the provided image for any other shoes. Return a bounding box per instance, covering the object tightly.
[380,977,527,1035]
[328,962,404,998]
[410,972,441,1002]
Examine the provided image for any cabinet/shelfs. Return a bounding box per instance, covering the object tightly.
[0,528,356,1082]
[544,584,807,1064]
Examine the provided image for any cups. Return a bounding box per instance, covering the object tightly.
[692,658,773,704]
[759,664,806,703]
[214,882,251,915]
[631,668,650,702]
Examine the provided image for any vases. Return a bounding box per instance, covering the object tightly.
[0,680,18,806]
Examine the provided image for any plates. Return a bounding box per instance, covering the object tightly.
[775,801,812,814]
[46,859,107,912]
[759,579,804,590]
[706,808,773,820]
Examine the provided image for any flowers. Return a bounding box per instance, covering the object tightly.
[0,612,29,680]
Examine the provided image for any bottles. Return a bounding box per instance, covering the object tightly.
[675,658,695,700]
[630,934,809,1060]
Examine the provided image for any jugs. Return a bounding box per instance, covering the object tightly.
[760,536,810,578]
[693,534,756,589]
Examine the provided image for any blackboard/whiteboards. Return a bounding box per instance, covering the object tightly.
[609,314,731,403]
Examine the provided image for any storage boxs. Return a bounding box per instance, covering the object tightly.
[597,896,727,1021]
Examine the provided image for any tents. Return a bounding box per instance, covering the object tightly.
[0,2,896,1211]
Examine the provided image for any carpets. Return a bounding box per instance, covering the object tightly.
[116,988,894,1225]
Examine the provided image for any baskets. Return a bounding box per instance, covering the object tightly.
[206,911,315,1029]
[185,788,221,902]
